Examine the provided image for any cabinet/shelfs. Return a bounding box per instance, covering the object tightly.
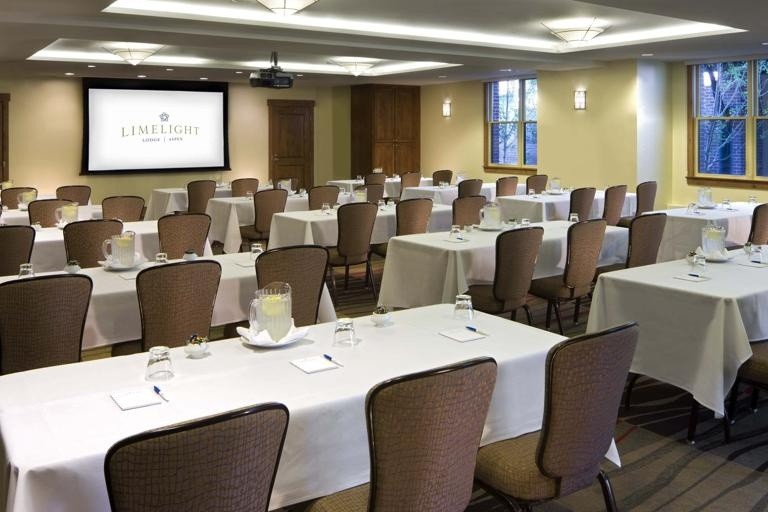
[352,85,420,179]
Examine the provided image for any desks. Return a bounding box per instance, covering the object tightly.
[0,302,571,511]
[585,202,768,443]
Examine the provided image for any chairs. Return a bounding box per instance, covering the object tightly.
[0,168,667,374]
[304,356,497,511]
[476,322,639,511]
[103,401,290,511]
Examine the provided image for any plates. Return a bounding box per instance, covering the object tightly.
[239,335,299,348]
[103,263,137,271]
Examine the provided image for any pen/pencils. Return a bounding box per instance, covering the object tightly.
[688,273,710,278]
[751,261,767,264]
[323,353,345,367]
[457,237,469,240]
[466,326,490,337]
[153,385,169,402]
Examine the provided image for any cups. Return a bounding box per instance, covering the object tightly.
[155,253,168,264]
[334,318,356,347]
[525,177,572,199]
[147,345,174,382]
[188,341,207,358]
[19,264,33,276]
[452,294,474,323]
[251,243,263,260]
[687,241,764,278]
[66,265,79,274]
[447,218,530,242]
[685,196,757,213]
[372,311,389,327]
[570,213,579,224]
[209,168,467,215]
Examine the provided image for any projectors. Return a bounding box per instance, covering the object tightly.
[249,69,293,89]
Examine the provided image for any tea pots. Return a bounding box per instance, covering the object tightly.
[55,202,79,223]
[701,227,726,256]
[16,190,37,207]
[102,230,136,266]
[0,180,13,191]
[479,204,500,227]
[698,188,713,207]
[248,281,292,341]
